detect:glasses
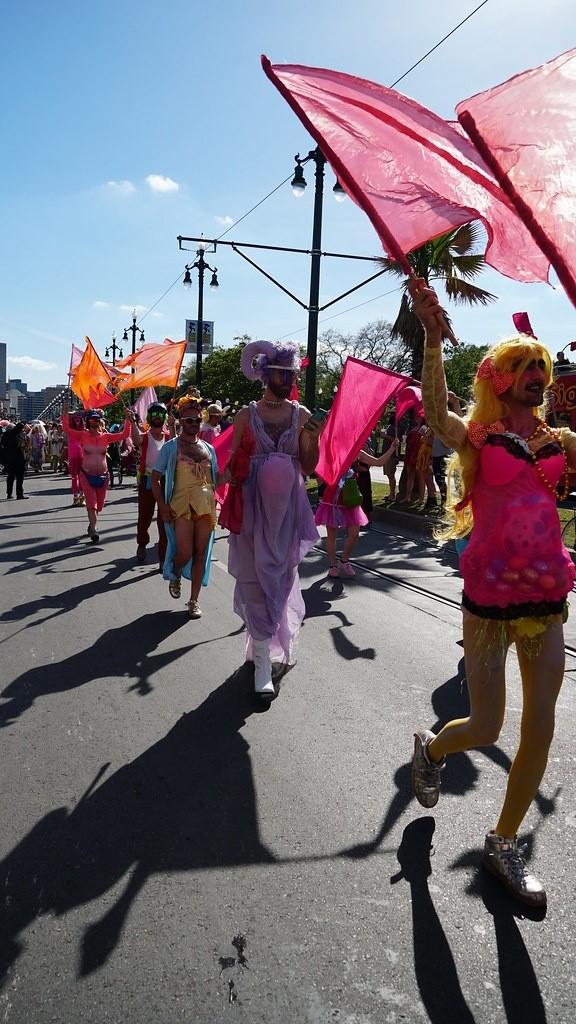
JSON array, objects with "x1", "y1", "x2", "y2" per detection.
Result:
[
  {"x1": 151, "y1": 412, "x2": 165, "y2": 418},
  {"x1": 182, "y1": 417, "x2": 203, "y2": 424}
]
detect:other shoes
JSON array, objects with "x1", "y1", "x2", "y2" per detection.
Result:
[
  {"x1": 17, "y1": 495, "x2": 28, "y2": 499},
  {"x1": 7, "y1": 495, "x2": 13, "y2": 499},
  {"x1": 383, "y1": 495, "x2": 445, "y2": 510},
  {"x1": 91, "y1": 533, "x2": 99, "y2": 542},
  {"x1": 73, "y1": 497, "x2": 80, "y2": 506},
  {"x1": 87, "y1": 526, "x2": 94, "y2": 533},
  {"x1": 79, "y1": 495, "x2": 86, "y2": 505},
  {"x1": 137, "y1": 545, "x2": 146, "y2": 561}
]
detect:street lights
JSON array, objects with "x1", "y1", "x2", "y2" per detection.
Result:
[
  {"x1": 37, "y1": 387, "x2": 79, "y2": 421},
  {"x1": 104, "y1": 331, "x2": 123, "y2": 366},
  {"x1": 290, "y1": 145, "x2": 347, "y2": 410},
  {"x1": 182, "y1": 232, "x2": 220, "y2": 384},
  {"x1": 122, "y1": 309, "x2": 145, "y2": 405}
]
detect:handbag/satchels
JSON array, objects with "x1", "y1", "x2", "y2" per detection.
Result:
[
  {"x1": 82, "y1": 468, "x2": 108, "y2": 486},
  {"x1": 341, "y1": 478, "x2": 363, "y2": 508}
]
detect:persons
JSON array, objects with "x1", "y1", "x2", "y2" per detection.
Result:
[
  {"x1": 410, "y1": 277, "x2": 576, "y2": 903},
  {"x1": 0, "y1": 390, "x2": 479, "y2": 511},
  {"x1": 151, "y1": 394, "x2": 228, "y2": 619},
  {"x1": 62, "y1": 402, "x2": 175, "y2": 572},
  {"x1": 224, "y1": 340, "x2": 324, "y2": 700},
  {"x1": 313, "y1": 437, "x2": 400, "y2": 579},
  {"x1": 553, "y1": 351, "x2": 570, "y2": 365}
]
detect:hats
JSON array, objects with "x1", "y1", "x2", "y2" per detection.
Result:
[{"x1": 207, "y1": 405, "x2": 225, "y2": 416}]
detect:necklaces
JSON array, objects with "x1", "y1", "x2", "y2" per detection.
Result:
[
  {"x1": 262, "y1": 395, "x2": 287, "y2": 409},
  {"x1": 505, "y1": 415, "x2": 568, "y2": 501},
  {"x1": 178, "y1": 434, "x2": 199, "y2": 445}
]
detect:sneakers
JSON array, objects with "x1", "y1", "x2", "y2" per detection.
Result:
[
  {"x1": 481, "y1": 830, "x2": 547, "y2": 905},
  {"x1": 169, "y1": 577, "x2": 181, "y2": 598},
  {"x1": 411, "y1": 727, "x2": 446, "y2": 808},
  {"x1": 329, "y1": 566, "x2": 339, "y2": 577},
  {"x1": 339, "y1": 559, "x2": 355, "y2": 576},
  {"x1": 185, "y1": 599, "x2": 201, "y2": 617}
]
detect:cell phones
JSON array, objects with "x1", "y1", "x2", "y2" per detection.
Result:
[{"x1": 303, "y1": 408, "x2": 328, "y2": 431}]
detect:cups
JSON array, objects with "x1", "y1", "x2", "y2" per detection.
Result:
[{"x1": 419, "y1": 426, "x2": 427, "y2": 433}]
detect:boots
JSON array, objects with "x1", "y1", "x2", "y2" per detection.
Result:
[{"x1": 252, "y1": 637, "x2": 275, "y2": 694}]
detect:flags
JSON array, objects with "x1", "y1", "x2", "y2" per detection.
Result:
[
  {"x1": 275, "y1": 47, "x2": 576, "y2": 317},
  {"x1": 314, "y1": 358, "x2": 425, "y2": 487},
  {"x1": 70, "y1": 334, "x2": 185, "y2": 409}
]
